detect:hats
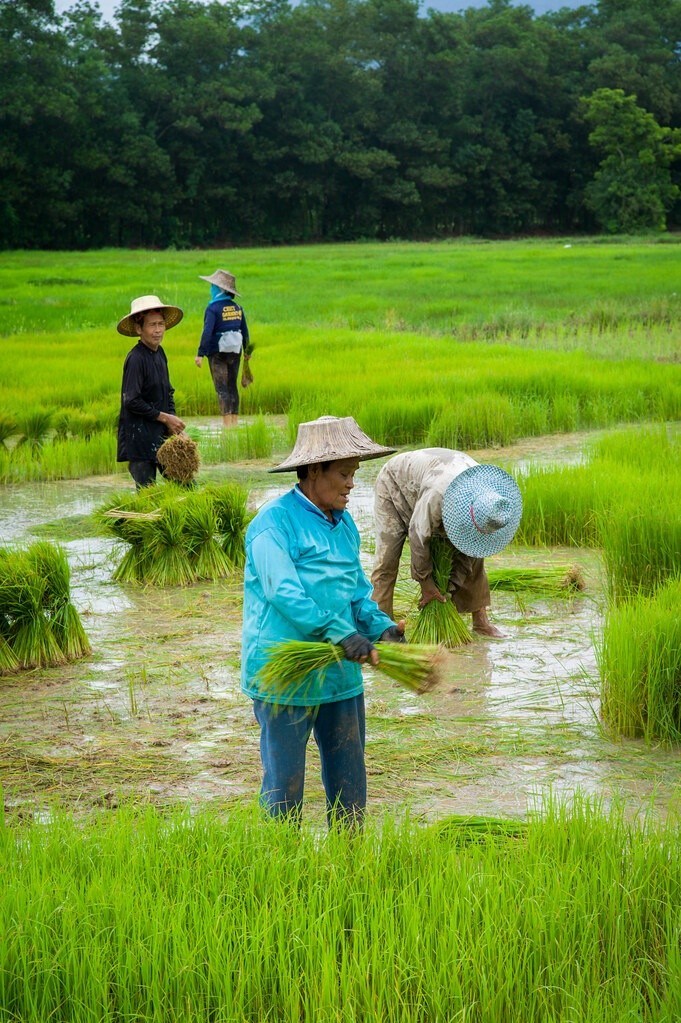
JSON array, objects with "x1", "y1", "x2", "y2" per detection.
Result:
[
  {"x1": 267, "y1": 415, "x2": 397, "y2": 474},
  {"x1": 117, "y1": 295, "x2": 183, "y2": 337},
  {"x1": 198, "y1": 269, "x2": 242, "y2": 299},
  {"x1": 441, "y1": 465, "x2": 522, "y2": 559}
]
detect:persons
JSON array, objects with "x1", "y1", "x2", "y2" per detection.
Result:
[
  {"x1": 371, "y1": 447, "x2": 523, "y2": 637},
  {"x1": 195, "y1": 270, "x2": 252, "y2": 427},
  {"x1": 240, "y1": 416, "x2": 409, "y2": 871},
  {"x1": 116, "y1": 295, "x2": 199, "y2": 496}
]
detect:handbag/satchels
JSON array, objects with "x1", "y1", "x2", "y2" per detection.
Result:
[{"x1": 218, "y1": 329, "x2": 243, "y2": 353}]
